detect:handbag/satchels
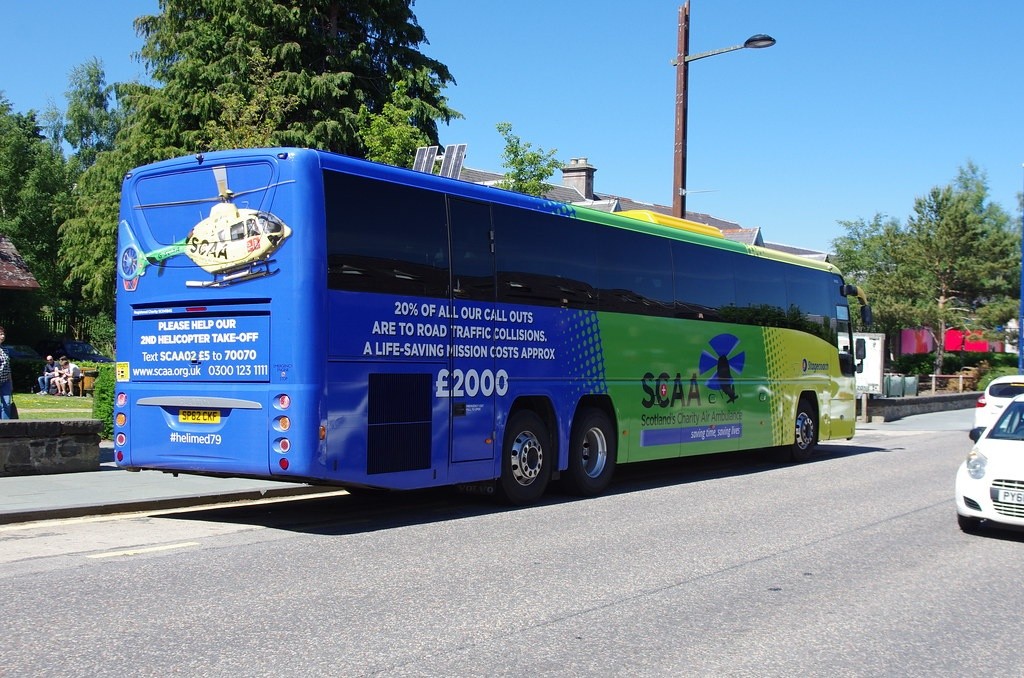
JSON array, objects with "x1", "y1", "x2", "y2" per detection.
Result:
[{"x1": 4, "y1": 402, "x2": 18, "y2": 419}]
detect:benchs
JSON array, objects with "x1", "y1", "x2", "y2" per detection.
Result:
[
  {"x1": 928, "y1": 374, "x2": 974, "y2": 395},
  {"x1": 73, "y1": 370, "x2": 85, "y2": 397}
]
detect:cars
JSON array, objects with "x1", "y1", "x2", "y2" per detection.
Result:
[
  {"x1": 975, "y1": 374, "x2": 1024, "y2": 446},
  {"x1": 954, "y1": 394, "x2": 1024, "y2": 534}
]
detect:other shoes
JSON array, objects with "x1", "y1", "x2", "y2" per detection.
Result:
[
  {"x1": 36, "y1": 391, "x2": 43, "y2": 395},
  {"x1": 55, "y1": 393, "x2": 62, "y2": 396},
  {"x1": 66, "y1": 392, "x2": 73, "y2": 397},
  {"x1": 62, "y1": 391, "x2": 67, "y2": 396},
  {"x1": 40, "y1": 391, "x2": 47, "y2": 395}
]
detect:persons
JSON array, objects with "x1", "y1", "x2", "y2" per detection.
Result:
[
  {"x1": 0, "y1": 326, "x2": 13, "y2": 419},
  {"x1": 50, "y1": 357, "x2": 68, "y2": 396},
  {"x1": 60, "y1": 361, "x2": 80, "y2": 397},
  {"x1": 36, "y1": 356, "x2": 62, "y2": 395}
]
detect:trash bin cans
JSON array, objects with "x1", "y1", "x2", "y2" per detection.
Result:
[{"x1": 883, "y1": 373, "x2": 919, "y2": 397}]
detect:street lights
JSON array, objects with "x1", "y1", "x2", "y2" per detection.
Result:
[{"x1": 671, "y1": 32, "x2": 778, "y2": 222}]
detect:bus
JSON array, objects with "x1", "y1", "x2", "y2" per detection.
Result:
[{"x1": 109, "y1": 146, "x2": 874, "y2": 505}]
